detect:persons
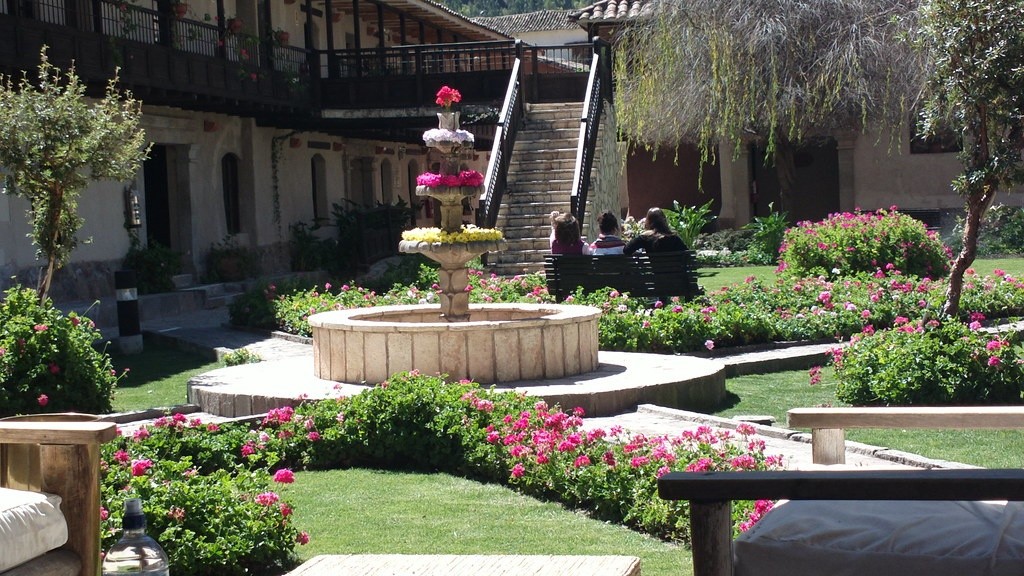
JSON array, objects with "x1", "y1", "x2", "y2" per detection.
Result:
[
  {"x1": 549, "y1": 211, "x2": 589, "y2": 256},
  {"x1": 587, "y1": 210, "x2": 659, "y2": 305},
  {"x1": 623, "y1": 207, "x2": 693, "y2": 308}
]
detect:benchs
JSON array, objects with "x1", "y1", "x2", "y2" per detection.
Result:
[{"x1": 542, "y1": 250, "x2": 706, "y2": 304}]
